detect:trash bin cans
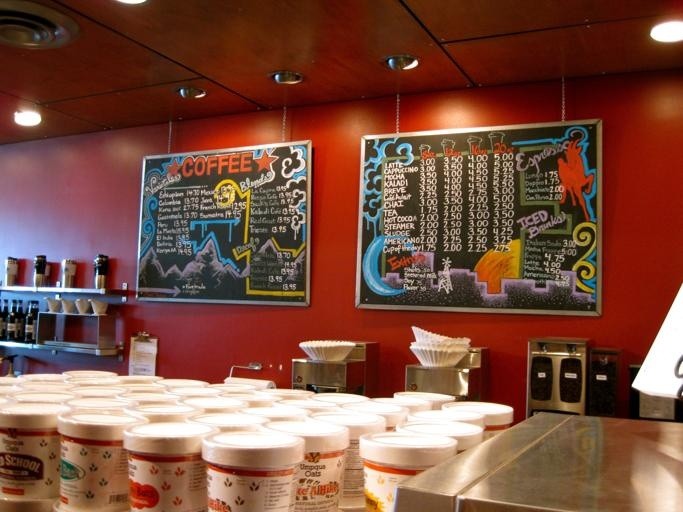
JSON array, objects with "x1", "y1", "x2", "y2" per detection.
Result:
[{"x1": 587, "y1": 350, "x2": 618, "y2": 416}]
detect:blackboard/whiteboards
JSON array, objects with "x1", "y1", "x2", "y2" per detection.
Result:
[
  {"x1": 135, "y1": 139, "x2": 313, "y2": 307},
  {"x1": 354, "y1": 118, "x2": 603, "y2": 318}
]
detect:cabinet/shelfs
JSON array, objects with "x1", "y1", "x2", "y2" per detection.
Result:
[{"x1": 0, "y1": 284, "x2": 127, "y2": 357}]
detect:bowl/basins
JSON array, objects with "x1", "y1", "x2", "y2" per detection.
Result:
[
  {"x1": 298, "y1": 340, "x2": 355, "y2": 363},
  {"x1": 43, "y1": 297, "x2": 110, "y2": 315},
  {"x1": 406, "y1": 325, "x2": 471, "y2": 368}
]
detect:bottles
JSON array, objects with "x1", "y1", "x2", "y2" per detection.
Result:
[{"x1": 0, "y1": 299, "x2": 39, "y2": 344}]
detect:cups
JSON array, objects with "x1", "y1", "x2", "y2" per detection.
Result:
[
  {"x1": 61, "y1": 258, "x2": 76, "y2": 288},
  {"x1": 33, "y1": 255, "x2": 50, "y2": 287},
  {"x1": 93, "y1": 253, "x2": 109, "y2": 290},
  {"x1": 4, "y1": 257, "x2": 17, "y2": 286}
]
